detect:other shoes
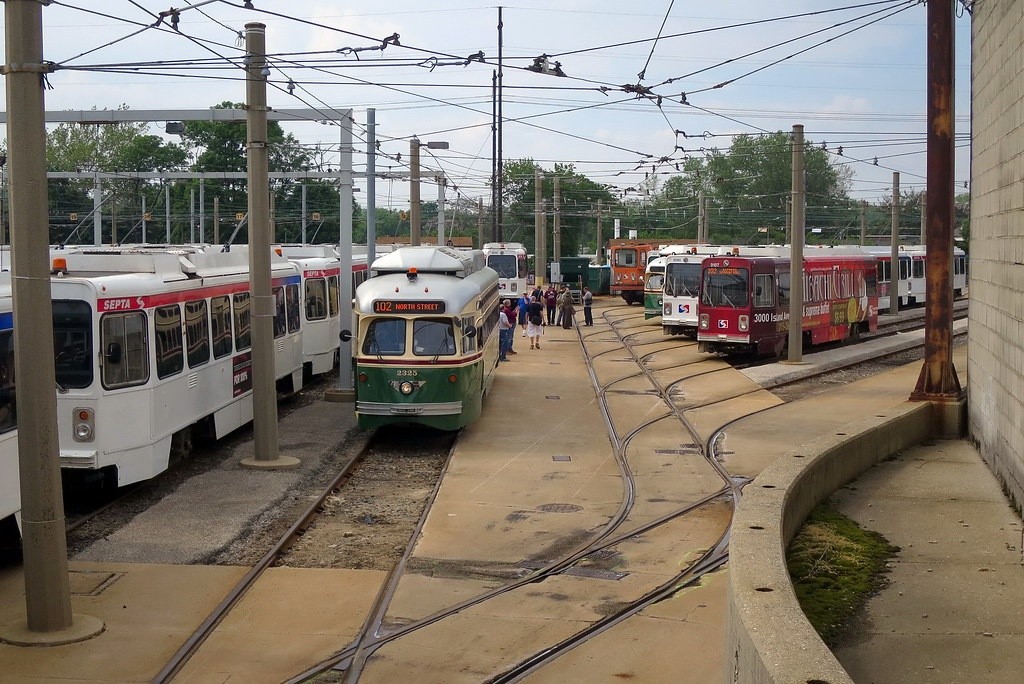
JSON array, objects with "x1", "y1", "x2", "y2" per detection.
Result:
[
  {"x1": 556, "y1": 323, "x2": 562, "y2": 326},
  {"x1": 500, "y1": 358, "x2": 510, "y2": 362},
  {"x1": 547, "y1": 320, "x2": 555, "y2": 325},
  {"x1": 506, "y1": 349, "x2": 517, "y2": 355},
  {"x1": 582, "y1": 323, "x2": 594, "y2": 326},
  {"x1": 529, "y1": 344, "x2": 534, "y2": 350},
  {"x1": 522, "y1": 330, "x2": 526, "y2": 337},
  {"x1": 564, "y1": 326, "x2": 571, "y2": 329},
  {"x1": 535, "y1": 342, "x2": 540, "y2": 350}
]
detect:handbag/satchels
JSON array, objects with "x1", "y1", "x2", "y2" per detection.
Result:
[
  {"x1": 585, "y1": 298, "x2": 592, "y2": 306},
  {"x1": 571, "y1": 307, "x2": 576, "y2": 315},
  {"x1": 531, "y1": 315, "x2": 542, "y2": 326}
]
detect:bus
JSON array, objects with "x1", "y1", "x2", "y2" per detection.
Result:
[
  {"x1": 608, "y1": 244, "x2": 965, "y2": 343},
  {"x1": 481, "y1": 240, "x2": 527, "y2": 304},
  {"x1": 696, "y1": 247, "x2": 879, "y2": 360},
  {"x1": 0, "y1": 241, "x2": 398, "y2": 547},
  {"x1": 340, "y1": 244, "x2": 502, "y2": 430}
]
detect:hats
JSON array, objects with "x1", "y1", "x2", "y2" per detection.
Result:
[
  {"x1": 548, "y1": 291, "x2": 555, "y2": 295},
  {"x1": 564, "y1": 291, "x2": 572, "y2": 297},
  {"x1": 522, "y1": 290, "x2": 528, "y2": 295}
]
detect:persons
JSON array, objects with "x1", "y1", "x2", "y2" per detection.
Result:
[
  {"x1": 544, "y1": 286, "x2": 557, "y2": 326},
  {"x1": 525, "y1": 296, "x2": 546, "y2": 349},
  {"x1": 503, "y1": 299, "x2": 517, "y2": 354},
  {"x1": 556, "y1": 285, "x2": 575, "y2": 329},
  {"x1": 532, "y1": 285, "x2": 544, "y2": 311},
  {"x1": 582, "y1": 287, "x2": 593, "y2": 326},
  {"x1": 516, "y1": 291, "x2": 531, "y2": 337},
  {"x1": 499, "y1": 304, "x2": 513, "y2": 362}
]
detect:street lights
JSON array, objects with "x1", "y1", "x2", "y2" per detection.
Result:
[{"x1": 411, "y1": 139, "x2": 450, "y2": 247}]
